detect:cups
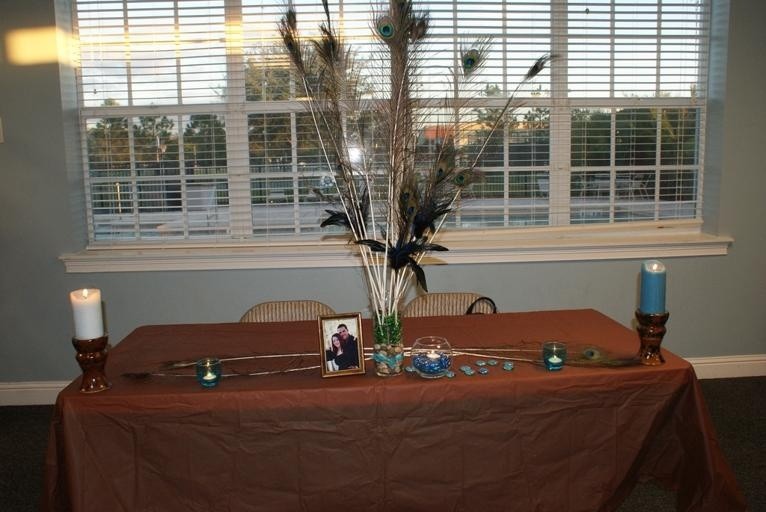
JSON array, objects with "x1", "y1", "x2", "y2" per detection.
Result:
[
  {"x1": 195, "y1": 358, "x2": 220, "y2": 387},
  {"x1": 542, "y1": 342, "x2": 566, "y2": 372}
]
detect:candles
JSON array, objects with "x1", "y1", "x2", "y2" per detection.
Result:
[
  {"x1": 69, "y1": 289, "x2": 103, "y2": 341},
  {"x1": 640, "y1": 259, "x2": 667, "y2": 315}
]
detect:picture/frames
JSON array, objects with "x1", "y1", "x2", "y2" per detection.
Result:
[{"x1": 316, "y1": 311, "x2": 367, "y2": 376}]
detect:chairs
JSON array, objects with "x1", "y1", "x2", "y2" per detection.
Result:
[
  {"x1": 403, "y1": 294, "x2": 491, "y2": 318},
  {"x1": 238, "y1": 301, "x2": 335, "y2": 323}
]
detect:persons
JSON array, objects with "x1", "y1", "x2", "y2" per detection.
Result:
[{"x1": 326, "y1": 324, "x2": 359, "y2": 370}]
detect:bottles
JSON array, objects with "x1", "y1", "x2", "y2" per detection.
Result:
[{"x1": 412, "y1": 336, "x2": 451, "y2": 378}]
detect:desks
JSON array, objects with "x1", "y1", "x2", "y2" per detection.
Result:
[{"x1": 50, "y1": 309, "x2": 740, "y2": 511}]
